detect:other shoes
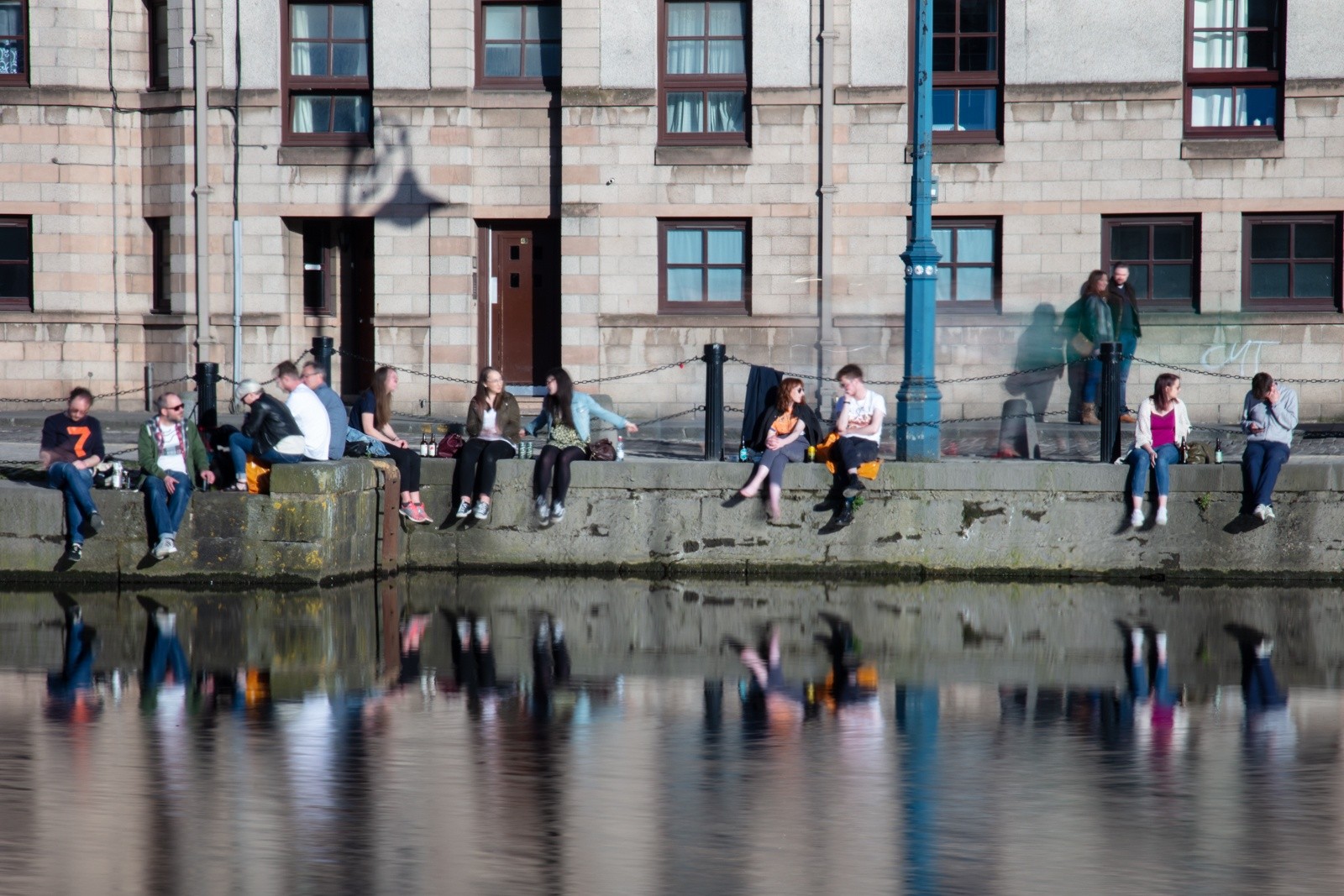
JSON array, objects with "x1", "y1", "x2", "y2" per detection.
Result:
[
  {"x1": 150, "y1": 540, "x2": 177, "y2": 559},
  {"x1": 1119, "y1": 412, "x2": 1136, "y2": 422},
  {"x1": 67, "y1": 545, "x2": 82, "y2": 561},
  {"x1": 536, "y1": 495, "x2": 549, "y2": 525},
  {"x1": 843, "y1": 481, "x2": 867, "y2": 499},
  {"x1": 222, "y1": 483, "x2": 249, "y2": 491},
  {"x1": 1155, "y1": 509, "x2": 1167, "y2": 526},
  {"x1": 90, "y1": 512, "x2": 105, "y2": 534},
  {"x1": 549, "y1": 500, "x2": 564, "y2": 523},
  {"x1": 1131, "y1": 509, "x2": 1145, "y2": 527},
  {"x1": 834, "y1": 508, "x2": 854, "y2": 526}
]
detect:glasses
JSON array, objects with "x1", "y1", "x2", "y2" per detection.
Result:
[
  {"x1": 792, "y1": 388, "x2": 803, "y2": 393},
  {"x1": 547, "y1": 378, "x2": 556, "y2": 385},
  {"x1": 165, "y1": 403, "x2": 184, "y2": 411}
]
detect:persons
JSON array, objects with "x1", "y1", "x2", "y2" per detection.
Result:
[
  {"x1": 301, "y1": 362, "x2": 348, "y2": 462},
  {"x1": 1107, "y1": 262, "x2": 1142, "y2": 423},
  {"x1": 726, "y1": 611, "x2": 883, "y2": 768},
  {"x1": 225, "y1": 380, "x2": 306, "y2": 492},
  {"x1": 1115, "y1": 373, "x2": 1191, "y2": 528},
  {"x1": 273, "y1": 361, "x2": 331, "y2": 462},
  {"x1": 739, "y1": 377, "x2": 823, "y2": 514},
  {"x1": 1245, "y1": 639, "x2": 1297, "y2": 764},
  {"x1": 829, "y1": 364, "x2": 886, "y2": 526},
  {"x1": 1077, "y1": 270, "x2": 1115, "y2": 425},
  {"x1": 454, "y1": 367, "x2": 521, "y2": 520},
  {"x1": 138, "y1": 392, "x2": 216, "y2": 559},
  {"x1": 348, "y1": 366, "x2": 434, "y2": 525},
  {"x1": 39, "y1": 386, "x2": 105, "y2": 562},
  {"x1": 1240, "y1": 372, "x2": 1298, "y2": 524},
  {"x1": 1132, "y1": 628, "x2": 1189, "y2": 758},
  {"x1": 397, "y1": 610, "x2": 572, "y2": 722},
  {"x1": 46, "y1": 605, "x2": 272, "y2": 731},
  {"x1": 521, "y1": 368, "x2": 638, "y2": 526}
]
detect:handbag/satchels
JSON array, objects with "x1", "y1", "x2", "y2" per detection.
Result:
[
  {"x1": 1073, "y1": 332, "x2": 1094, "y2": 357},
  {"x1": 345, "y1": 426, "x2": 390, "y2": 456},
  {"x1": 438, "y1": 433, "x2": 465, "y2": 457},
  {"x1": 587, "y1": 439, "x2": 616, "y2": 461},
  {"x1": 244, "y1": 452, "x2": 270, "y2": 493},
  {"x1": 1185, "y1": 442, "x2": 1214, "y2": 464}
]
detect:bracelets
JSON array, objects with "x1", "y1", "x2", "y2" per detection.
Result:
[
  {"x1": 393, "y1": 437, "x2": 398, "y2": 440},
  {"x1": 843, "y1": 401, "x2": 850, "y2": 404}
]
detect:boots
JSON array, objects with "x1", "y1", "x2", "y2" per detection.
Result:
[{"x1": 1080, "y1": 401, "x2": 1100, "y2": 425}]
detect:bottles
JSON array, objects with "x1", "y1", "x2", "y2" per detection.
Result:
[
  {"x1": 616, "y1": 435, "x2": 625, "y2": 460},
  {"x1": 1216, "y1": 438, "x2": 1223, "y2": 464},
  {"x1": 428, "y1": 433, "x2": 437, "y2": 457},
  {"x1": 739, "y1": 435, "x2": 747, "y2": 462},
  {"x1": 1181, "y1": 436, "x2": 1188, "y2": 464},
  {"x1": 420, "y1": 433, "x2": 428, "y2": 458},
  {"x1": 808, "y1": 446, "x2": 815, "y2": 463}
]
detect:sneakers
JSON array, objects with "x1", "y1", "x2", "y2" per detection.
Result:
[
  {"x1": 456, "y1": 501, "x2": 472, "y2": 517},
  {"x1": 475, "y1": 502, "x2": 489, "y2": 520},
  {"x1": 1254, "y1": 504, "x2": 1275, "y2": 524},
  {"x1": 415, "y1": 502, "x2": 433, "y2": 524},
  {"x1": 398, "y1": 502, "x2": 427, "y2": 523}
]
